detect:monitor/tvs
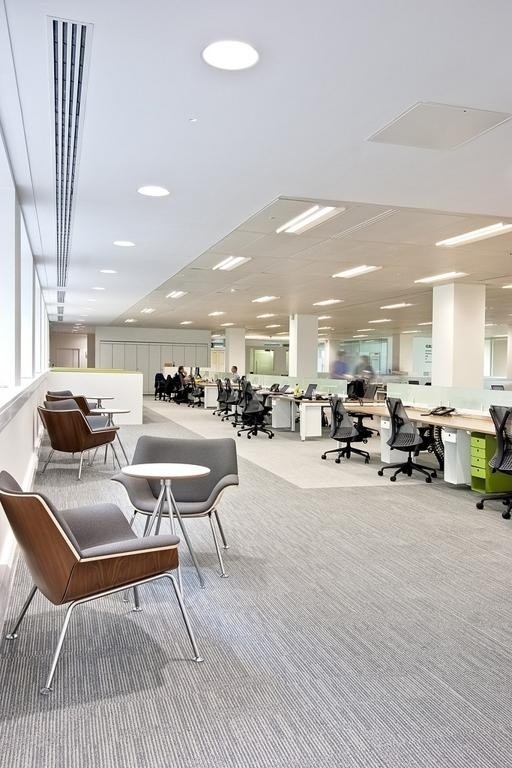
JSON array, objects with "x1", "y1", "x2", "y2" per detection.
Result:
[{"x1": 190, "y1": 366, "x2": 201, "y2": 379}]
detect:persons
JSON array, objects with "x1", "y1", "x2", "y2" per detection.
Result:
[
  {"x1": 354, "y1": 353, "x2": 375, "y2": 374},
  {"x1": 174, "y1": 365, "x2": 188, "y2": 388},
  {"x1": 329, "y1": 348, "x2": 351, "y2": 379},
  {"x1": 228, "y1": 364, "x2": 240, "y2": 391}
]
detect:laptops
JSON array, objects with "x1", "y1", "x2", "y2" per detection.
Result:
[
  {"x1": 270, "y1": 385, "x2": 290, "y2": 395},
  {"x1": 294, "y1": 384, "x2": 317, "y2": 400}
]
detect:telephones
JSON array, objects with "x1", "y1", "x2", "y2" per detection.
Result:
[
  {"x1": 270, "y1": 383, "x2": 279, "y2": 392},
  {"x1": 431, "y1": 407, "x2": 455, "y2": 416},
  {"x1": 309, "y1": 394, "x2": 321, "y2": 400}
]
